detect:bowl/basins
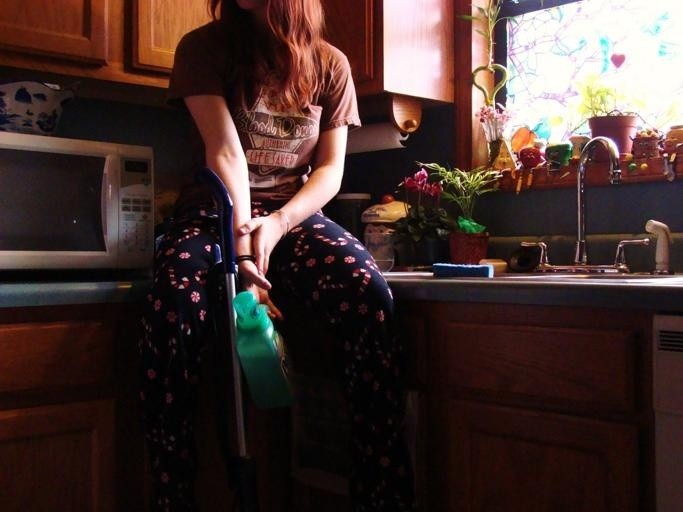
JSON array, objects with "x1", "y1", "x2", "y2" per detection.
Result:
[{"x1": 0, "y1": 82, "x2": 76, "y2": 134}]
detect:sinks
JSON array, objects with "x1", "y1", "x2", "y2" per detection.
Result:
[{"x1": 570, "y1": 272, "x2": 673, "y2": 281}]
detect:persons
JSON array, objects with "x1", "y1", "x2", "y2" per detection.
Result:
[{"x1": 136, "y1": 0, "x2": 417, "y2": 510}]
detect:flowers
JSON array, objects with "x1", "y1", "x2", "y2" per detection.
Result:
[
  {"x1": 393, "y1": 164, "x2": 449, "y2": 240},
  {"x1": 477, "y1": 103, "x2": 511, "y2": 121}
]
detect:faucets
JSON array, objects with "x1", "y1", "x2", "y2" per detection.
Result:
[{"x1": 520, "y1": 136, "x2": 651, "y2": 272}]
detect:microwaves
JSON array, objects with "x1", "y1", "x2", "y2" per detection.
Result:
[{"x1": 0, "y1": 132, "x2": 157, "y2": 272}]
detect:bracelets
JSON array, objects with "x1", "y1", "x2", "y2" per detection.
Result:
[
  {"x1": 269, "y1": 209, "x2": 290, "y2": 235},
  {"x1": 234, "y1": 257, "x2": 255, "y2": 266},
  {"x1": 232, "y1": 254, "x2": 256, "y2": 262}
]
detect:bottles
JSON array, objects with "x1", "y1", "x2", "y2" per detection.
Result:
[{"x1": 334, "y1": 192, "x2": 371, "y2": 243}]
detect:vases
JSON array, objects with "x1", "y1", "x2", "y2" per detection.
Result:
[
  {"x1": 483, "y1": 119, "x2": 505, "y2": 167},
  {"x1": 415, "y1": 239, "x2": 445, "y2": 266}
]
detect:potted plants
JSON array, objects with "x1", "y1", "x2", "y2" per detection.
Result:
[
  {"x1": 417, "y1": 161, "x2": 504, "y2": 265},
  {"x1": 559, "y1": 79, "x2": 676, "y2": 153}
]
